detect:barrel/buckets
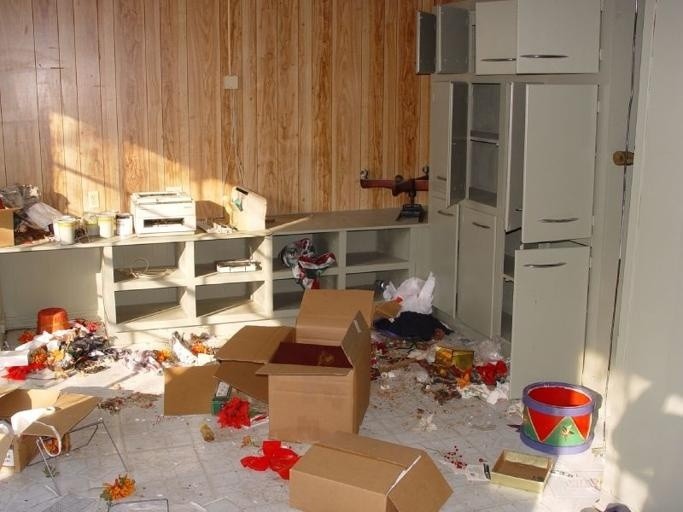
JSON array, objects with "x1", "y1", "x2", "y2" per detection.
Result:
[{"x1": 37, "y1": 308, "x2": 71, "y2": 330}]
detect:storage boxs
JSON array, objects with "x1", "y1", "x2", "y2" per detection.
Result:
[
  {"x1": 1, "y1": 388, "x2": 104, "y2": 472},
  {"x1": 163, "y1": 326, "x2": 268, "y2": 416},
  {"x1": 289, "y1": 430, "x2": 452, "y2": 511},
  {"x1": 214, "y1": 289, "x2": 402, "y2": 446}
]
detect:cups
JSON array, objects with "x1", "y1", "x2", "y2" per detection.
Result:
[
  {"x1": 52, "y1": 218, "x2": 76, "y2": 246},
  {"x1": 116, "y1": 215, "x2": 134, "y2": 235},
  {"x1": 97, "y1": 217, "x2": 114, "y2": 237},
  {"x1": 86, "y1": 223, "x2": 98, "y2": 235}
]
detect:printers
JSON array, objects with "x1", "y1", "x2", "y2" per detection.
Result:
[{"x1": 130, "y1": 188, "x2": 197, "y2": 238}]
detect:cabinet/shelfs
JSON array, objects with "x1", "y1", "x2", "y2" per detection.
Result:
[{"x1": 1, "y1": 0, "x2": 642, "y2": 399}]
